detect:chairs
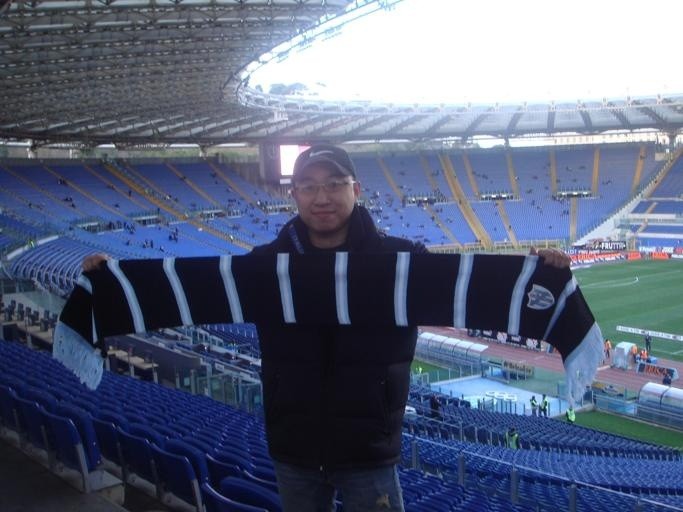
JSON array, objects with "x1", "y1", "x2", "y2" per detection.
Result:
[{"x1": 0, "y1": 337, "x2": 681, "y2": 512}]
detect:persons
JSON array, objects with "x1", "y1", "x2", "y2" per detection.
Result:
[
  {"x1": 57, "y1": 170, "x2": 294, "y2": 253},
  {"x1": 633, "y1": 333, "x2": 652, "y2": 363},
  {"x1": 566, "y1": 405, "x2": 576, "y2": 425},
  {"x1": 604, "y1": 339, "x2": 612, "y2": 358},
  {"x1": 504, "y1": 428, "x2": 521, "y2": 451},
  {"x1": 662, "y1": 374, "x2": 669, "y2": 386},
  {"x1": 530, "y1": 394, "x2": 548, "y2": 418},
  {"x1": 361, "y1": 162, "x2": 612, "y2": 245},
  {"x1": 82, "y1": 145, "x2": 572, "y2": 512},
  {"x1": 429, "y1": 394, "x2": 441, "y2": 418}
]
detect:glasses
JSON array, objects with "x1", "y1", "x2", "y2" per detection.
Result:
[{"x1": 291, "y1": 179, "x2": 358, "y2": 191}]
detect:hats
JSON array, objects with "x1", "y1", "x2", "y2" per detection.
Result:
[{"x1": 293, "y1": 144, "x2": 356, "y2": 177}]
